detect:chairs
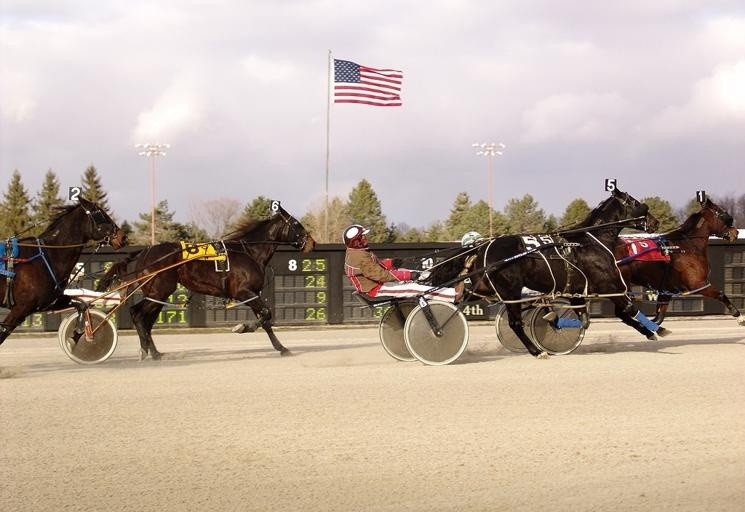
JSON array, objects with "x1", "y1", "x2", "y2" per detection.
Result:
[{"x1": 352, "y1": 290, "x2": 405, "y2": 330}]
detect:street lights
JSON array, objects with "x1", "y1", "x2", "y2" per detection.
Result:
[
  {"x1": 471, "y1": 143, "x2": 505, "y2": 236},
  {"x1": 132, "y1": 142, "x2": 173, "y2": 245}
]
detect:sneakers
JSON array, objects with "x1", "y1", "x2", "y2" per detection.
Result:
[
  {"x1": 455, "y1": 281, "x2": 465, "y2": 302},
  {"x1": 119, "y1": 284, "x2": 127, "y2": 303}
]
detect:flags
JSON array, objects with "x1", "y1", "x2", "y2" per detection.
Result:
[{"x1": 335, "y1": 59, "x2": 404, "y2": 108}]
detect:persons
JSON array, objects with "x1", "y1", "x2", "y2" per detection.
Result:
[
  {"x1": 342, "y1": 223, "x2": 472, "y2": 306},
  {"x1": 63, "y1": 283, "x2": 130, "y2": 306},
  {"x1": 459, "y1": 229, "x2": 543, "y2": 296}
]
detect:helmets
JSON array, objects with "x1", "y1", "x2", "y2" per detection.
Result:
[
  {"x1": 461, "y1": 231, "x2": 481, "y2": 248},
  {"x1": 342, "y1": 225, "x2": 370, "y2": 248}
]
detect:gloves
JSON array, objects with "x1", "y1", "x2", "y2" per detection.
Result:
[
  {"x1": 410, "y1": 271, "x2": 420, "y2": 281},
  {"x1": 393, "y1": 259, "x2": 403, "y2": 267}
]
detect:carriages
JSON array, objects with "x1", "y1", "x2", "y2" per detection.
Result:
[
  {"x1": 36, "y1": 205, "x2": 317, "y2": 366},
  {"x1": 352, "y1": 188, "x2": 674, "y2": 367},
  {"x1": 493, "y1": 187, "x2": 744, "y2": 367}
]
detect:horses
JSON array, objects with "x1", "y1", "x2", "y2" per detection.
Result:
[
  {"x1": 611, "y1": 198, "x2": 745, "y2": 327},
  {"x1": 92, "y1": 204, "x2": 316, "y2": 361},
  {"x1": 0, "y1": 194, "x2": 129, "y2": 347},
  {"x1": 431, "y1": 186, "x2": 673, "y2": 360}
]
